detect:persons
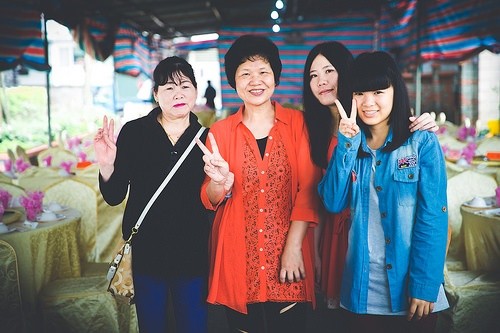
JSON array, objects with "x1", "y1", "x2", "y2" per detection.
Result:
[
  {"x1": 195, "y1": 34, "x2": 320, "y2": 333},
  {"x1": 316, "y1": 50, "x2": 452, "y2": 333},
  {"x1": 302, "y1": 39, "x2": 441, "y2": 332},
  {"x1": 202, "y1": 78, "x2": 217, "y2": 109},
  {"x1": 91, "y1": 55, "x2": 235, "y2": 333}
]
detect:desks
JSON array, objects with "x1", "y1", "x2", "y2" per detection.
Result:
[
  {"x1": 460, "y1": 197, "x2": 500, "y2": 271},
  {"x1": 0, "y1": 208, "x2": 97, "y2": 292}
]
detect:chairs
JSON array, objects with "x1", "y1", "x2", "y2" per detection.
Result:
[
  {"x1": 0, "y1": 179, "x2": 97, "y2": 233},
  {"x1": 444, "y1": 170, "x2": 500, "y2": 333}
]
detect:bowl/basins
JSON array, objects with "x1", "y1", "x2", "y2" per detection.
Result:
[
  {"x1": 40, "y1": 211, "x2": 56, "y2": 220},
  {"x1": 469, "y1": 197, "x2": 486, "y2": 206},
  {"x1": 48, "y1": 202, "x2": 62, "y2": 211},
  {"x1": 0, "y1": 222, "x2": 9, "y2": 233}
]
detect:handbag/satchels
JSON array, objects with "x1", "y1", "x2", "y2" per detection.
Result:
[{"x1": 105, "y1": 240, "x2": 135, "y2": 306}]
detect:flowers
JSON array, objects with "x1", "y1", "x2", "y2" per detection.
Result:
[{"x1": 0, "y1": 153, "x2": 86, "y2": 221}]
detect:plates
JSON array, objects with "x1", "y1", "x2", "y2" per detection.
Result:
[
  {"x1": 463, "y1": 200, "x2": 495, "y2": 208},
  {"x1": 488, "y1": 208, "x2": 500, "y2": 216},
  {"x1": 36, "y1": 214, "x2": 66, "y2": 222},
  {"x1": 0, "y1": 226, "x2": 16, "y2": 234},
  {"x1": 42, "y1": 206, "x2": 68, "y2": 213}
]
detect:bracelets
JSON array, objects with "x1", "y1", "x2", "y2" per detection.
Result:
[{"x1": 224, "y1": 194, "x2": 232, "y2": 198}]
